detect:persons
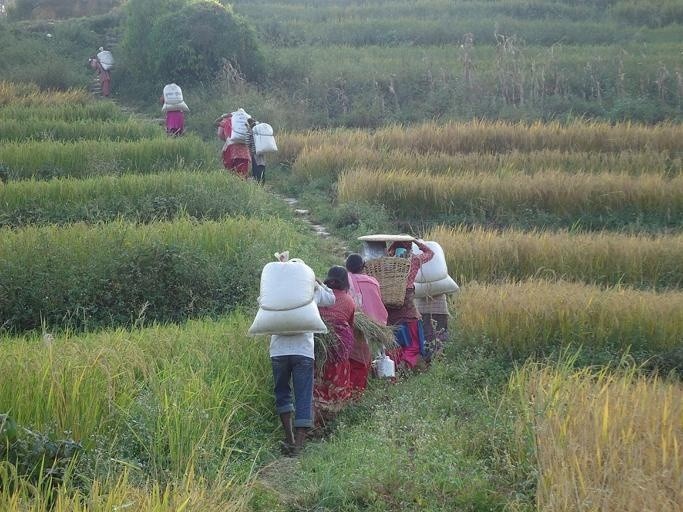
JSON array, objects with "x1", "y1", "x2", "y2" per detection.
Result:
[
  {"x1": 381, "y1": 232, "x2": 435, "y2": 385},
  {"x1": 416, "y1": 294, "x2": 450, "y2": 359},
  {"x1": 245, "y1": 117, "x2": 267, "y2": 187},
  {"x1": 160, "y1": 95, "x2": 185, "y2": 135},
  {"x1": 213, "y1": 113, "x2": 252, "y2": 181},
  {"x1": 89, "y1": 55, "x2": 111, "y2": 97},
  {"x1": 311, "y1": 264, "x2": 356, "y2": 428},
  {"x1": 269, "y1": 276, "x2": 336, "y2": 458},
  {"x1": 345, "y1": 254, "x2": 388, "y2": 401}
]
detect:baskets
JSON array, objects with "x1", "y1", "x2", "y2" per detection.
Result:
[{"x1": 363, "y1": 256, "x2": 410, "y2": 308}]
